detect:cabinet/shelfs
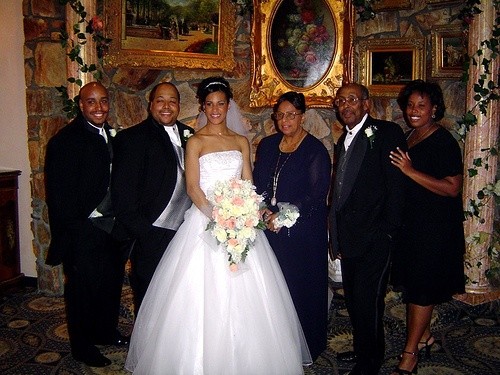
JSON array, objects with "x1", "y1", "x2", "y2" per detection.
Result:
[{"x1": 0, "y1": 170, "x2": 25, "y2": 293}]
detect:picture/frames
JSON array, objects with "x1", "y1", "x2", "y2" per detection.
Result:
[
  {"x1": 103, "y1": 0, "x2": 236, "y2": 71},
  {"x1": 357, "y1": 38, "x2": 426, "y2": 99},
  {"x1": 431, "y1": 24, "x2": 468, "y2": 78},
  {"x1": 249, "y1": 0, "x2": 357, "y2": 110}
]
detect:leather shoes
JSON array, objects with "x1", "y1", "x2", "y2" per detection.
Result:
[
  {"x1": 115, "y1": 336, "x2": 129, "y2": 346},
  {"x1": 349, "y1": 358, "x2": 385, "y2": 375},
  {"x1": 337, "y1": 351, "x2": 357, "y2": 362},
  {"x1": 72, "y1": 350, "x2": 112, "y2": 367}
]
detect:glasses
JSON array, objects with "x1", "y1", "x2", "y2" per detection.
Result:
[
  {"x1": 274, "y1": 111, "x2": 303, "y2": 120},
  {"x1": 334, "y1": 97, "x2": 366, "y2": 107}
]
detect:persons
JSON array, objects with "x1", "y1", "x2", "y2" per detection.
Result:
[
  {"x1": 328, "y1": 81, "x2": 409, "y2": 375},
  {"x1": 44, "y1": 81, "x2": 130, "y2": 367},
  {"x1": 125, "y1": 77, "x2": 314, "y2": 375},
  {"x1": 252, "y1": 91, "x2": 332, "y2": 362},
  {"x1": 109, "y1": 82, "x2": 196, "y2": 322}
]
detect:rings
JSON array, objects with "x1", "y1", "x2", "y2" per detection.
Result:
[{"x1": 400, "y1": 157, "x2": 402, "y2": 160}]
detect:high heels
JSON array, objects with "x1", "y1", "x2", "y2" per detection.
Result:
[
  {"x1": 417, "y1": 335, "x2": 436, "y2": 355},
  {"x1": 394, "y1": 351, "x2": 419, "y2": 375}
]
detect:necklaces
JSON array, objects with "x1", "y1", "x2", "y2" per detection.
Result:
[
  {"x1": 409, "y1": 128, "x2": 432, "y2": 144},
  {"x1": 270, "y1": 134, "x2": 303, "y2": 206},
  {"x1": 390, "y1": 79, "x2": 466, "y2": 375},
  {"x1": 207, "y1": 127, "x2": 225, "y2": 136}
]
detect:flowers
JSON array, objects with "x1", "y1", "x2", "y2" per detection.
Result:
[
  {"x1": 365, "y1": 125, "x2": 378, "y2": 142},
  {"x1": 183, "y1": 129, "x2": 193, "y2": 142},
  {"x1": 109, "y1": 129, "x2": 117, "y2": 137},
  {"x1": 206, "y1": 177, "x2": 268, "y2": 272},
  {"x1": 55, "y1": 0, "x2": 112, "y2": 121}
]
